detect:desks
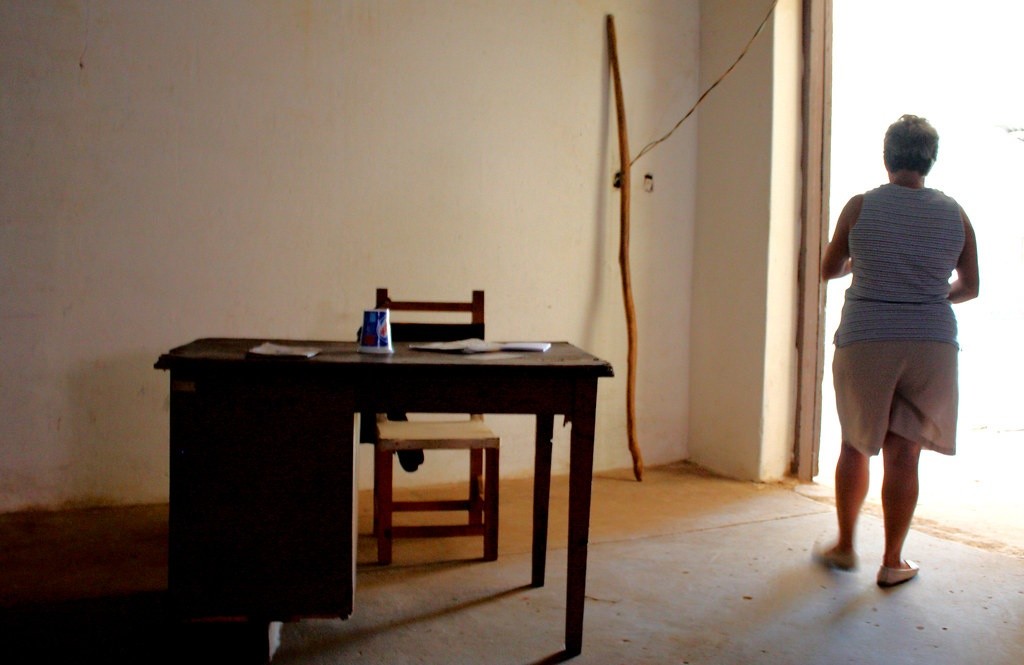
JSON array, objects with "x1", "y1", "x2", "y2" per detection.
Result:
[{"x1": 155, "y1": 337, "x2": 615, "y2": 658}]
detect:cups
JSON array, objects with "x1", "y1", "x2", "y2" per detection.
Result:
[{"x1": 357, "y1": 309, "x2": 393, "y2": 353}]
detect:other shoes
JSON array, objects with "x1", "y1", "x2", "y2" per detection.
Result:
[
  {"x1": 815, "y1": 542, "x2": 856, "y2": 570},
  {"x1": 877, "y1": 559, "x2": 918, "y2": 587}
]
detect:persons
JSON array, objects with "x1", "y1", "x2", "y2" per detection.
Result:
[{"x1": 815, "y1": 115, "x2": 980, "y2": 586}]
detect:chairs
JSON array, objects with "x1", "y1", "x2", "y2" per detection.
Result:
[{"x1": 373, "y1": 287, "x2": 501, "y2": 566}]
